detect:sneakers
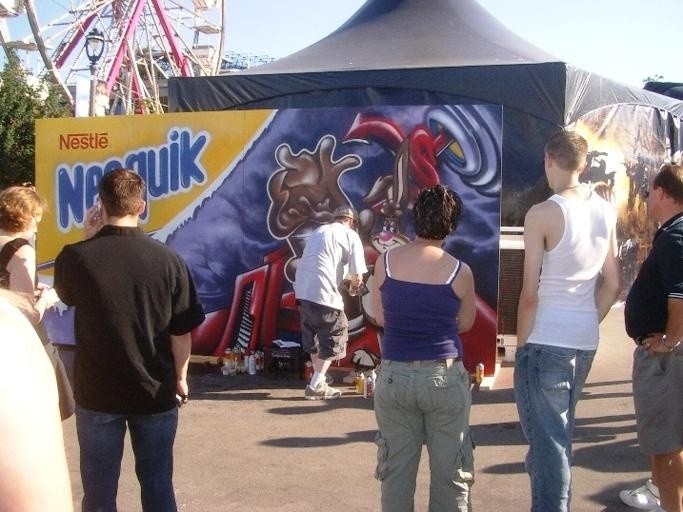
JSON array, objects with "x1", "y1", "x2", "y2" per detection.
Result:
[
  {"x1": 305, "y1": 372, "x2": 341, "y2": 400},
  {"x1": 619, "y1": 479, "x2": 666, "y2": 512}
]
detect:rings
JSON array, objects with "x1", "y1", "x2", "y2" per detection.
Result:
[{"x1": 646, "y1": 342, "x2": 651, "y2": 347}]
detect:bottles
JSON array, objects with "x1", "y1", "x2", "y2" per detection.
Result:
[
  {"x1": 475, "y1": 362, "x2": 484, "y2": 383},
  {"x1": 356, "y1": 371, "x2": 373, "y2": 398},
  {"x1": 304, "y1": 359, "x2": 312, "y2": 381},
  {"x1": 221, "y1": 346, "x2": 265, "y2": 376}
]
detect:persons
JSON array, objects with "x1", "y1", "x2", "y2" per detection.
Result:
[
  {"x1": 54, "y1": 170, "x2": 205, "y2": 512},
  {"x1": 372, "y1": 183, "x2": 475, "y2": 512},
  {"x1": 0, "y1": 186, "x2": 74, "y2": 512},
  {"x1": 294, "y1": 204, "x2": 369, "y2": 401},
  {"x1": 513, "y1": 131, "x2": 620, "y2": 512},
  {"x1": 620, "y1": 163, "x2": 682, "y2": 512}
]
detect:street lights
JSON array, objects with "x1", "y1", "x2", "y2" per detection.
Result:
[{"x1": 84, "y1": 28, "x2": 104, "y2": 117}]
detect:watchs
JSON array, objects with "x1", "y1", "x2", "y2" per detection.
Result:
[{"x1": 661, "y1": 334, "x2": 680, "y2": 350}]
